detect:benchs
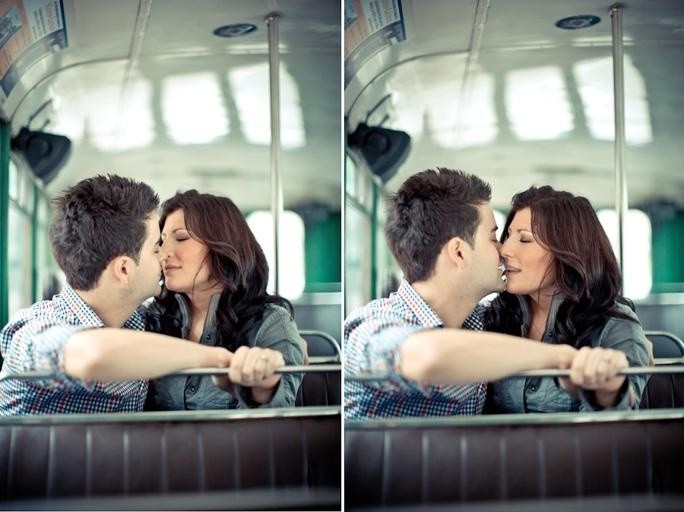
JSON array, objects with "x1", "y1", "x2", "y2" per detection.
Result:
[
  {"x1": 0, "y1": 323, "x2": 341, "y2": 511},
  {"x1": 344, "y1": 331, "x2": 683, "y2": 511}
]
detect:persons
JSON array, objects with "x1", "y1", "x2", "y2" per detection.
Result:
[
  {"x1": 144, "y1": 190, "x2": 306, "y2": 410},
  {"x1": 343, "y1": 166, "x2": 654, "y2": 417},
  {"x1": 484, "y1": 185, "x2": 650, "y2": 414},
  {"x1": 0, "y1": 173, "x2": 308, "y2": 415}
]
